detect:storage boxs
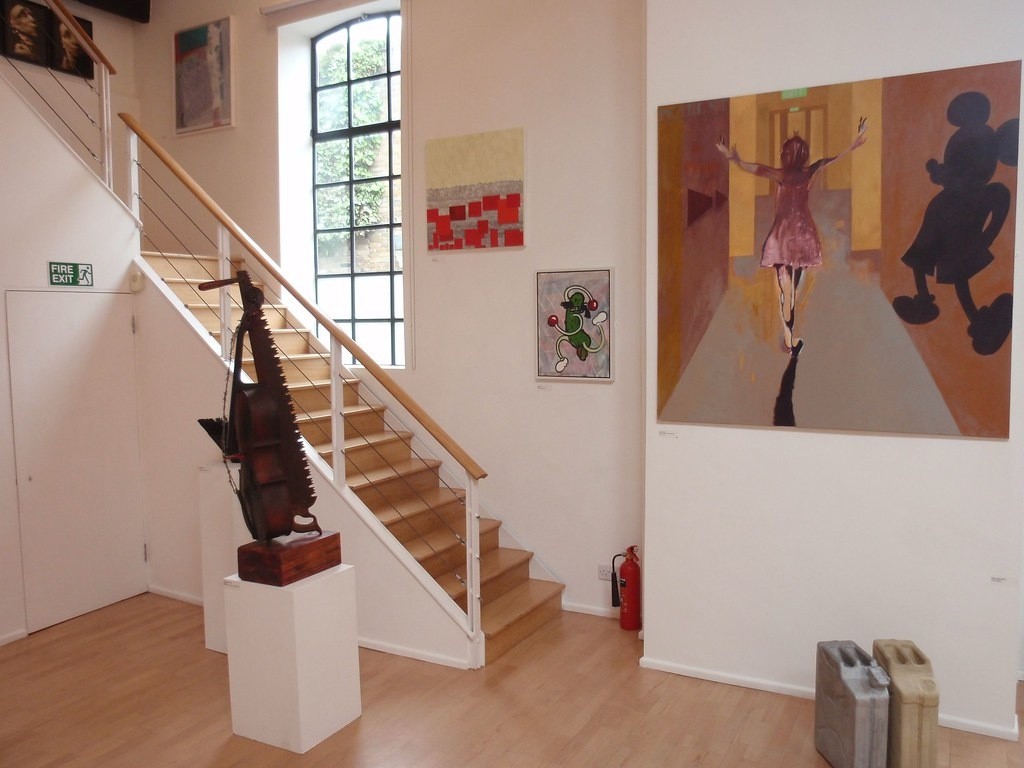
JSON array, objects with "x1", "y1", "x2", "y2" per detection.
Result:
[{"x1": 238, "y1": 530, "x2": 341, "y2": 587}]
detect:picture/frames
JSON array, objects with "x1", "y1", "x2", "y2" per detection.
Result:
[
  {"x1": 0, "y1": 0, "x2": 94, "y2": 80},
  {"x1": 174, "y1": 15, "x2": 236, "y2": 138},
  {"x1": 535, "y1": 266, "x2": 616, "y2": 382}
]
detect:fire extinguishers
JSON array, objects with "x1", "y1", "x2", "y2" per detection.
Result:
[{"x1": 611, "y1": 545, "x2": 641, "y2": 631}]
